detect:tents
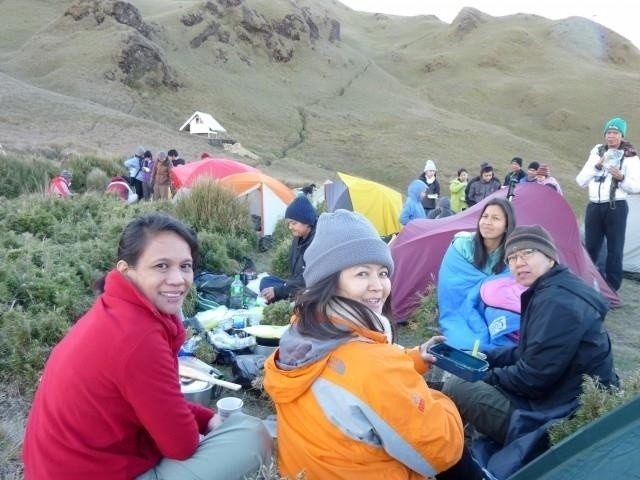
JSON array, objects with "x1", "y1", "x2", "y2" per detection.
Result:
[
  {"x1": 313, "y1": 172, "x2": 409, "y2": 244},
  {"x1": 216, "y1": 172, "x2": 296, "y2": 237},
  {"x1": 384, "y1": 182, "x2": 622, "y2": 324},
  {"x1": 580, "y1": 193, "x2": 640, "y2": 282},
  {"x1": 169, "y1": 158, "x2": 258, "y2": 203}
]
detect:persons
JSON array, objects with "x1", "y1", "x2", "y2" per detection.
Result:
[
  {"x1": 504, "y1": 157, "x2": 527, "y2": 186},
  {"x1": 536, "y1": 164, "x2": 564, "y2": 196},
  {"x1": 262, "y1": 209, "x2": 483, "y2": 480},
  {"x1": 576, "y1": 118, "x2": 640, "y2": 292},
  {"x1": 260, "y1": 197, "x2": 318, "y2": 305},
  {"x1": 47, "y1": 169, "x2": 73, "y2": 199},
  {"x1": 520, "y1": 161, "x2": 540, "y2": 183},
  {"x1": 420, "y1": 159, "x2": 440, "y2": 216},
  {"x1": 25, "y1": 214, "x2": 273, "y2": 480},
  {"x1": 465, "y1": 162, "x2": 500, "y2": 207},
  {"x1": 106, "y1": 146, "x2": 186, "y2": 205},
  {"x1": 441, "y1": 224, "x2": 619, "y2": 445},
  {"x1": 428, "y1": 196, "x2": 455, "y2": 219},
  {"x1": 399, "y1": 179, "x2": 429, "y2": 225},
  {"x1": 297, "y1": 183, "x2": 316, "y2": 199},
  {"x1": 437, "y1": 198, "x2": 530, "y2": 352},
  {"x1": 449, "y1": 168, "x2": 469, "y2": 214}
]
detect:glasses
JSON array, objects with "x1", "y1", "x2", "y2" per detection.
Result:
[{"x1": 505, "y1": 247, "x2": 538, "y2": 266}]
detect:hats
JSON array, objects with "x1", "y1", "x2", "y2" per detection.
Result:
[
  {"x1": 285, "y1": 196, "x2": 316, "y2": 226},
  {"x1": 111, "y1": 176, "x2": 125, "y2": 181},
  {"x1": 528, "y1": 161, "x2": 539, "y2": 171},
  {"x1": 511, "y1": 157, "x2": 522, "y2": 166},
  {"x1": 303, "y1": 209, "x2": 394, "y2": 288},
  {"x1": 136, "y1": 147, "x2": 144, "y2": 156},
  {"x1": 604, "y1": 118, "x2": 626, "y2": 138},
  {"x1": 536, "y1": 164, "x2": 550, "y2": 176},
  {"x1": 423, "y1": 160, "x2": 436, "y2": 172},
  {"x1": 505, "y1": 224, "x2": 560, "y2": 265},
  {"x1": 60, "y1": 170, "x2": 72, "y2": 178}
]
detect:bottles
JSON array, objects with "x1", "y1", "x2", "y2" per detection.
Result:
[
  {"x1": 230, "y1": 273, "x2": 244, "y2": 309},
  {"x1": 232, "y1": 311, "x2": 247, "y2": 339}
]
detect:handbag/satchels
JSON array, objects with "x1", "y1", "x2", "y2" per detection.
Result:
[{"x1": 130, "y1": 162, "x2": 143, "y2": 186}]
